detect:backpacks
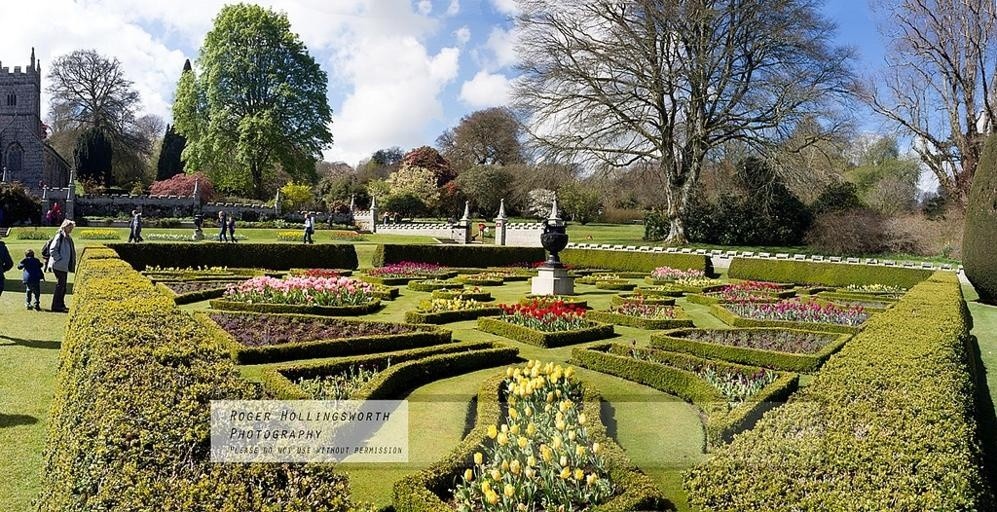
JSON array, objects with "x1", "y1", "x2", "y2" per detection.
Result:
[{"x1": 41, "y1": 234, "x2": 63, "y2": 257}]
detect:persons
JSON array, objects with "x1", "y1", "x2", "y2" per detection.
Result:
[
  {"x1": 133, "y1": 211, "x2": 143, "y2": 243},
  {"x1": 128, "y1": 210, "x2": 140, "y2": 242},
  {"x1": 46, "y1": 218, "x2": 76, "y2": 312},
  {"x1": 0, "y1": 241, "x2": 13, "y2": 295},
  {"x1": 383, "y1": 212, "x2": 389, "y2": 225},
  {"x1": 46, "y1": 202, "x2": 63, "y2": 226},
  {"x1": 217, "y1": 215, "x2": 228, "y2": 242},
  {"x1": 17, "y1": 249, "x2": 45, "y2": 311},
  {"x1": 303, "y1": 214, "x2": 313, "y2": 244},
  {"x1": 393, "y1": 212, "x2": 401, "y2": 225},
  {"x1": 228, "y1": 217, "x2": 237, "y2": 243}
]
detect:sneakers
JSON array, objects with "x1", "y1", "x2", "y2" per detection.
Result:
[
  {"x1": 34, "y1": 305, "x2": 41, "y2": 311},
  {"x1": 26, "y1": 304, "x2": 32, "y2": 309}
]
